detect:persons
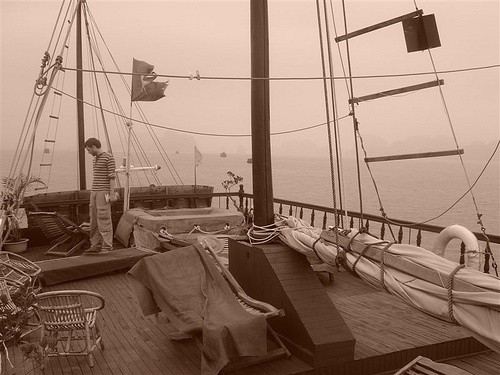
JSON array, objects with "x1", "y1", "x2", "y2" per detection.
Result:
[{"x1": 82, "y1": 138, "x2": 119, "y2": 255}]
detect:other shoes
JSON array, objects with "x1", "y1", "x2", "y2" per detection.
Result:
[
  {"x1": 84, "y1": 248, "x2": 102, "y2": 253},
  {"x1": 100, "y1": 247, "x2": 114, "y2": 254}
]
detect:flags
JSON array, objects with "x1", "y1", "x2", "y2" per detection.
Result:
[{"x1": 131, "y1": 57, "x2": 168, "y2": 102}]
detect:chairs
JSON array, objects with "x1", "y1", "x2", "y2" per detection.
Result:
[
  {"x1": 26, "y1": 290, "x2": 107, "y2": 369},
  {"x1": 27, "y1": 212, "x2": 94, "y2": 258},
  {"x1": 0, "y1": 250, "x2": 43, "y2": 327}
]
